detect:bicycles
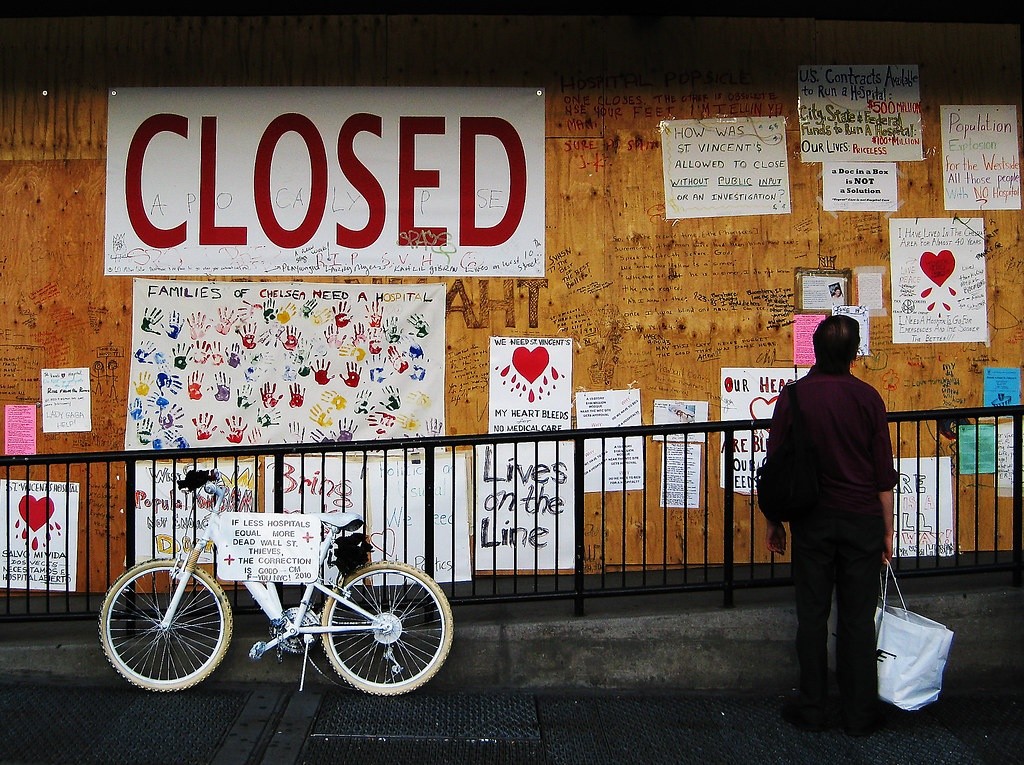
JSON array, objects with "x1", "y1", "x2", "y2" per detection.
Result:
[{"x1": 96, "y1": 467, "x2": 456, "y2": 699}]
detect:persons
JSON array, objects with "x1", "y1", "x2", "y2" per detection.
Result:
[
  {"x1": 757, "y1": 315, "x2": 899, "y2": 737},
  {"x1": 832, "y1": 287, "x2": 843, "y2": 299}
]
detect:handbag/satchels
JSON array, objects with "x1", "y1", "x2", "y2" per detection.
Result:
[
  {"x1": 757, "y1": 381, "x2": 819, "y2": 523},
  {"x1": 827, "y1": 558, "x2": 954, "y2": 711}
]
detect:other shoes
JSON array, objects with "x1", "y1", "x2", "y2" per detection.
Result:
[
  {"x1": 782, "y1": 701, "x2": 825, "y2": 731},
  {"x1": 845, "y1": 713, "x2": 886, "y2": 736}
]
